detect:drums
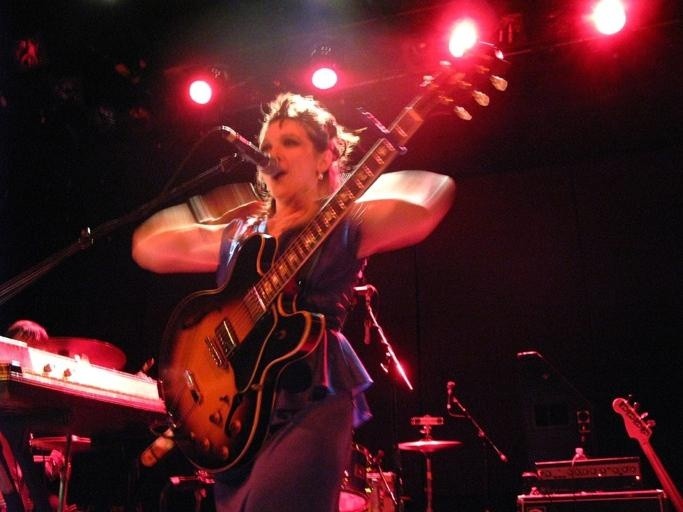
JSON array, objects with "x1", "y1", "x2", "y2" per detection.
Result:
[{"x1": 338, "y1": 443, "x2": 398, "y2": 512}]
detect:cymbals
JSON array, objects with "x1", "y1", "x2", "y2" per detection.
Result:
[
  {"x1": 29, "y1": 333, "x2": 128, "y2": 370},
  {"x1": 397, "y1": 440, "x2": 463, "y2": 451},
  {"x1": 30, "y1": 437, "x2": 92, "y2": 453}
]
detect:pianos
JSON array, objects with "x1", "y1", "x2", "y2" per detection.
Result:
[
  {"x1": 516, "y1": 487, "x2": 665, "y2": 508},
  {"x1": 534, "y1": 457, "x2": 642, "y2": 481},
  {"x1": 0, "y1": 335, "x2": 166, "y2": 421}
]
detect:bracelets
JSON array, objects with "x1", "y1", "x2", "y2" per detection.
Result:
[{"x1": 187, "y1": 194, "x2": 209, "y2": 223}]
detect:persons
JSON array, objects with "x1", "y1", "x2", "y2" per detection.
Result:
[
  {"x1": 130, "y1": 90, "x2": 458, "y2": 511},
  {"x1": 6, "y1": 319, "x2": 51, "y2": 345}
]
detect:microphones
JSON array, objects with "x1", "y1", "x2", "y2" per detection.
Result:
[
  {"x1": 515, "y1": 348, "x2": 545, "y2": 364},
  {"x1": 446, "y1": 381, "x2": 455, "y2": 409},
  {"x1": 221, "y1": 126, "x2": 280, "y2": 176}
]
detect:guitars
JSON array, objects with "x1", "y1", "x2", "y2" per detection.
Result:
[
  {"x1": 612, "y1": 398, "x2": 683, "y2": 509},
  {"x1": 160, "y1": 42, "x2": 514, "y2": 481}
]
